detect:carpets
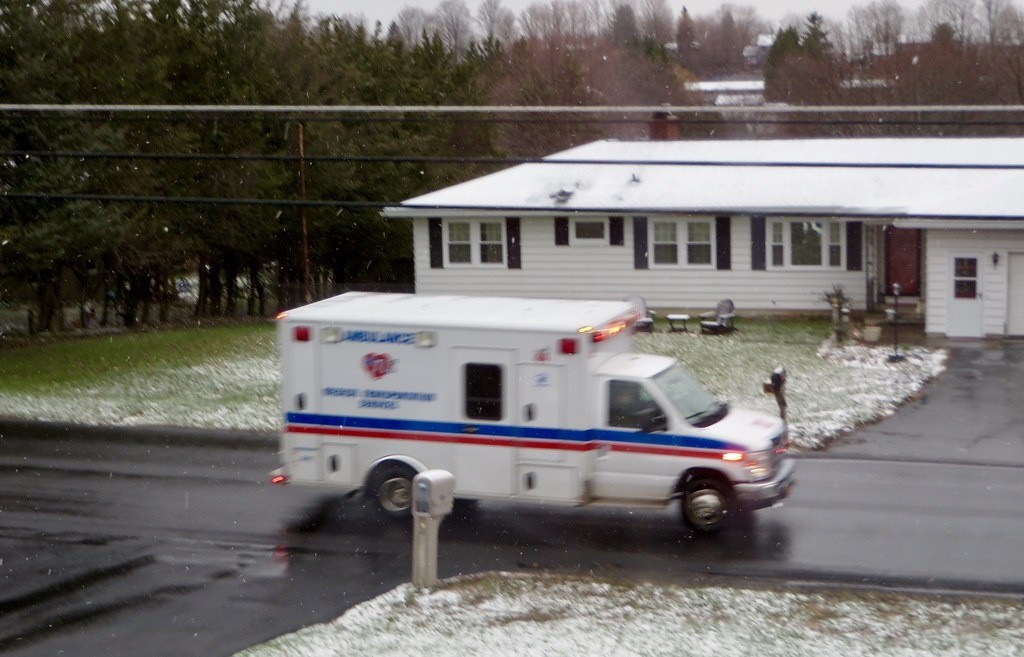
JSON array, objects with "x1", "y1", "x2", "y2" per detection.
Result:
[{"x1": 890, "y1": 302, "x2": 917, "y2": 307}]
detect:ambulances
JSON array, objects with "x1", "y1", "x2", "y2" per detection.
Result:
[{"x1": 268, "y1": 289, "x2": 798, "y2": 539}]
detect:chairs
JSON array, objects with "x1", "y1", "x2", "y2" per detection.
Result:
[
  {"x1": 623, "y1": 295, "x2": 656, "y2": 332},
  {"x1": 698, "y1": 299, "x2": 736, "y2": 333}
]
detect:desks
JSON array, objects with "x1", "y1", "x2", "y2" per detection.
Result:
[{"x1": 666, "y1": 314, "x2": 690, "y2": 331}]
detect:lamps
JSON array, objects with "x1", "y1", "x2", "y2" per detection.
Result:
[{"x1": 992, "y1": 251, "x2": 1000, "y2": 264}]
